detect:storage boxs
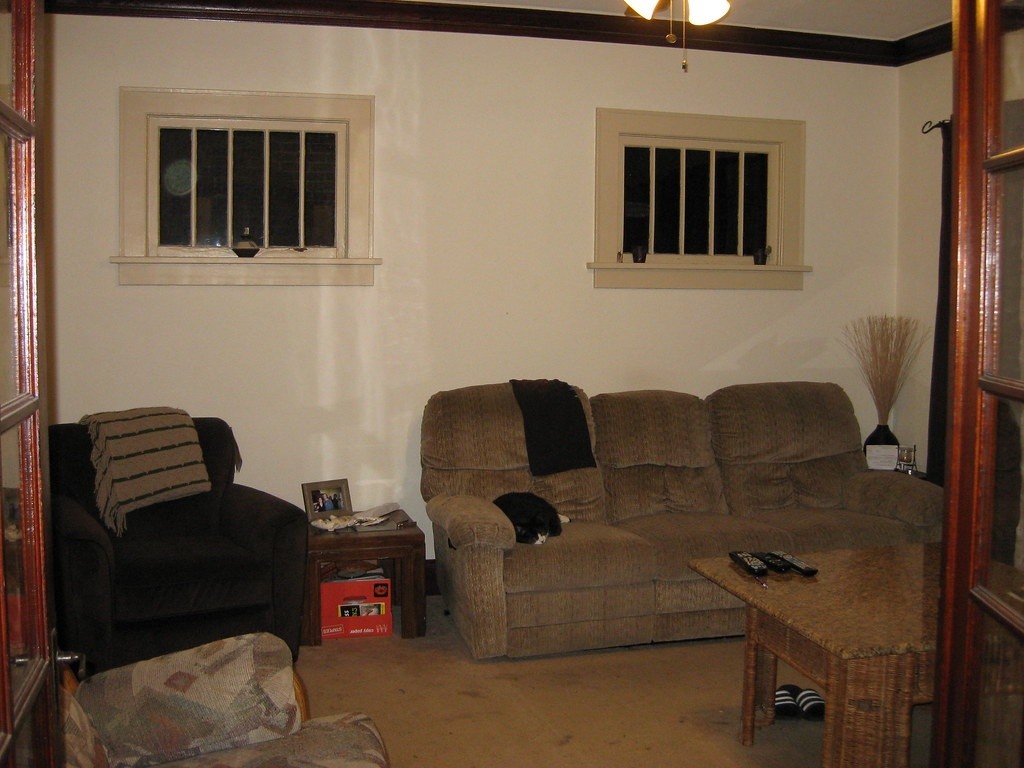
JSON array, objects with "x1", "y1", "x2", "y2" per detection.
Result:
[{"x1": 319, "y1": 573, "x2": 395, "y2": 641}]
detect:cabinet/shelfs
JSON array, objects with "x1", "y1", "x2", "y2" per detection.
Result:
[{"x1": 929, "y1": 0, "x2": 1024, "y2": 768}]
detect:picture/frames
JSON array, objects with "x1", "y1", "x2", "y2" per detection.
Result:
[{"x1": 301, "y1": 477, "x2": 356, "y2": 520}]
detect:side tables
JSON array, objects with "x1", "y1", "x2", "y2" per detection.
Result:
[{"x1": 302, "y1": 508, "x2": 426, "y2": 647}]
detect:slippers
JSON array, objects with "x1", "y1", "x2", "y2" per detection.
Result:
[{"x1": 776, "y1": 683, "x2": 825, "y2": 721}]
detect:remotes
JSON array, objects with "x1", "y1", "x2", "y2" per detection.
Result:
[
  {"x1": 771, "y1": 550, "x2": 818, "y2": 576},
  {"x1": 729, "y1": 551, "x2": 768, "y2": 575},
  {"x1": 751, "y1": 552, "x2": 791, "y2": 573}
]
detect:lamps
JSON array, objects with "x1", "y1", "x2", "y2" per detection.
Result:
[{"x1": 619, "y1": 0, "x2": 736, "y2": 74}]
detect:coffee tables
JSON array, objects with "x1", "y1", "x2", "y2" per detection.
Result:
[{"x1": 684, "y1": 543, "x2": 948, "y2": 768}]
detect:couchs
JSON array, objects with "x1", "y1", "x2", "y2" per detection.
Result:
[
  {"x1": 59, "y1": 629, "x2": 389, "y2": 768},
  {"x1": 45, "y1": 418, "x2": 308, "y2": 681},
  {"x1": 419, "y1": 380, "x2": 946, "y2": 662}
]
